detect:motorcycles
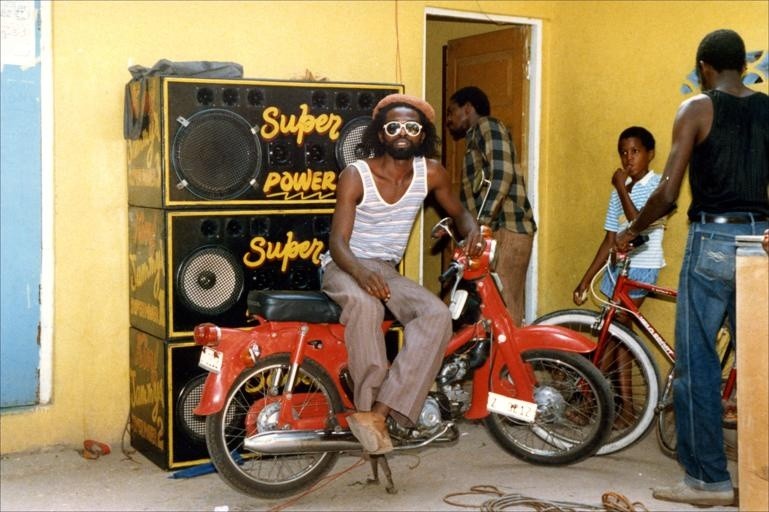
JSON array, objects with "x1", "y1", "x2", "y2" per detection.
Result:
[{"x1": 188, "y1": 166, "x2": 619, "y2": 502}]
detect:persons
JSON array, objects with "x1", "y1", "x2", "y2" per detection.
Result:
[
  {"x1": 614, "y1": 30, "x2": 769, "y2": 506},
  {"x1": 565, "y1": 127, "x2": 667, "y2": 431},
  {"x1": 320, "y1": 92, "x2": 488, "y2": 457},
  {"x1": 446, "y1": 87, "x2": 537, "y2": 326}
]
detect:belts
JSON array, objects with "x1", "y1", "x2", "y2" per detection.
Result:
[{"x1": 689, "y1": 214, "x2": 769, "y2": 224}]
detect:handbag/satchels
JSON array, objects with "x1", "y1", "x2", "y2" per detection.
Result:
[{"x1": 128, "y1": 60, "x2": 243, "y2": 79}]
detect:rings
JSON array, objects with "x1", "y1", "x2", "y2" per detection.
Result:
[{"x1": 477, "y1": 241, "x2": 482, "y2": 247}]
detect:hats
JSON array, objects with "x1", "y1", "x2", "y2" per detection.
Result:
[{"x1": 373, "y1": 93, "x2": 435, "y2": 125}]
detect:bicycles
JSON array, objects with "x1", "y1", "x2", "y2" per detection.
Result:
[{"x1": 513, "y1": 203, "x2": 737, "y2": 459}]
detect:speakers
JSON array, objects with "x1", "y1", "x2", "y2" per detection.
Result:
[
  {"x1": 128, "y1": 206, "x2": 404, "y2": 341},
  {"x1": 128, "y1": 326, "x2": 402, "y2": 470},
  {"x1": 125, "y1": 76, "x2": 404, "y2": 210}
]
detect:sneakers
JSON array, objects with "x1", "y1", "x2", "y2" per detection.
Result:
[
  {"x1": 346, "y1": 412, "x2": 392, "y2": 455},
  {"x1": 653, "y1": 481, "x2": 734, "y2": 506}
]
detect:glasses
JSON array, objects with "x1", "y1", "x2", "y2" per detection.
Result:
[{"x1": 384, "y1": 121, "x2": 423, "y2": 137}]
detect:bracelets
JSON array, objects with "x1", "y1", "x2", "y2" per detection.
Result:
[{"x1": 625, "y1": 226, "x2": 639, "y2": 237}]
